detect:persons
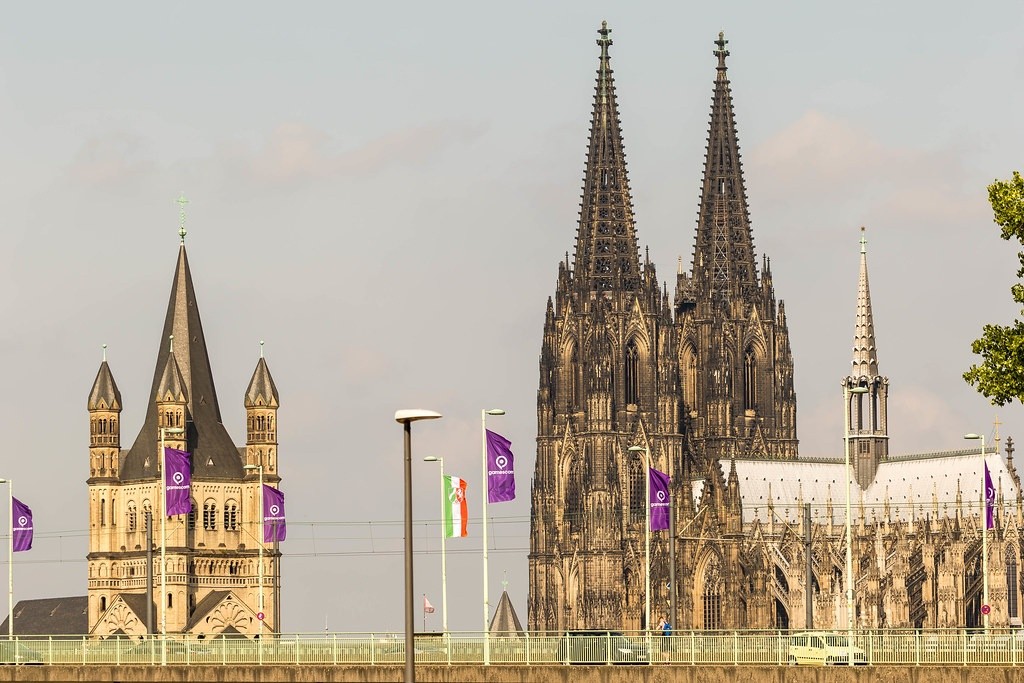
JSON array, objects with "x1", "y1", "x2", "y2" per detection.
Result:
[{"x1": 656, "y1": 618, "x2": 671, "y2": 661}]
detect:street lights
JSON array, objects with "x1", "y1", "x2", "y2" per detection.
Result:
[
  {"x1": 242, "y1": 464, "x2": 264, "y2": 636},
  {"x1": 394, "y1": 409, "x2": 444, "y2": 683},
  {"x1": 0, "y1": 478, "x2": 14, "y2": 642},
  {"x1": 423, "y1": 455, "x2": 448, "y2": 643},
  {"x1": 963, "y1": 432, "x2": 991, "y2": 635},
  {"x1": 844, "y1": 386, "x2": 870, "y2": 667},
  {"x1": 159, "y1": 427, "x2": 184, "y2": 666},
  {"x1": 627, "y1": 444, "x2": 652, "y2": 645},
  {"x1": 481, "y1": 408, "x2": 506, "y2": 666}
]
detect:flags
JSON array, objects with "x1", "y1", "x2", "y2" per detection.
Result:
[
  {"x1": 442, "y1": 474, "x2": 468, "y2": 538},
  {"x1": 485, "y1": 428, "x2": 516, "y2": 503},
  {"x1": 261, "y1": 484, "x2": 286, "y2": 543},
  {"x1": 424, "y1": 598, "x2": 435, "y2": 613},
  {"x1": 647, "y1": 468, "x2": 670, "y2": 531},
  {"x1": 162, "y1": 447, "x2": 192, "y2": 516},
  {"x1": 982, "y1": 460, "x2": 993, "y2": 530},
  {"x1": 11, "y1": 496, "x2": 33, "y2": 552}
]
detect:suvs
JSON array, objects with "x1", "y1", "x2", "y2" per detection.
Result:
[
  {"x1": 788, "y1": 633, "x2": 869, "y2": 665},
  {"x1": 554, "y1": 628, "x2": 650, "y2": 665}
]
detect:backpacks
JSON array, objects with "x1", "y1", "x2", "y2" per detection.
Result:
[{"x1": 662, "y1": 621, "x2": 672, "y2": 636}]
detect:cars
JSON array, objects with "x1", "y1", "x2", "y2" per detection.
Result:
[
  {"x1": 124, "y1": 639, "x2": 211, "y2": 655},
  {"x1": 0, "y1": 641, "x2": 43, "y2": 662},
  {"x1": 385, "y1": 644, "x2": 428, "y2": 655}
]
